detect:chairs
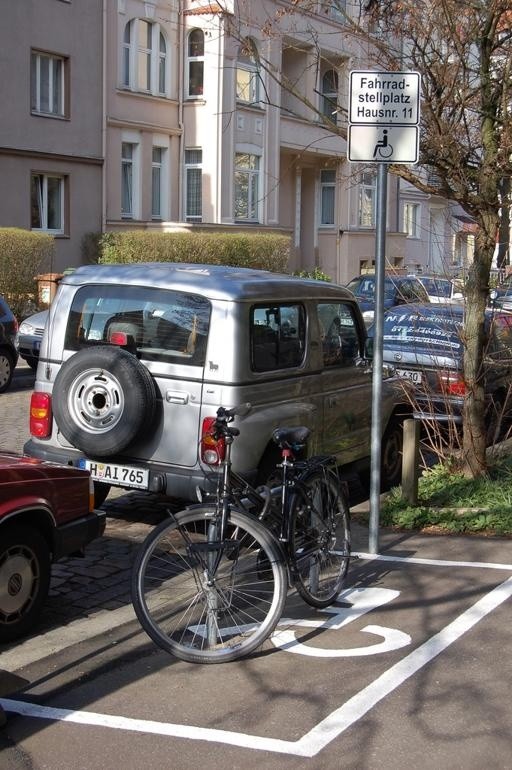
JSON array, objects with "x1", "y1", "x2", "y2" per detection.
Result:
[
  {"x1": 251, "y1": 326, "x2": 277, "y2": 344},
  {"x1": 104, "y1": 314, "x2": 143, "y2": 346}
]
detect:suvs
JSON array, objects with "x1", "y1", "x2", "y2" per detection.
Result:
[{"x1": 25, "y1": 261, "x2": 418, "y2": 505}]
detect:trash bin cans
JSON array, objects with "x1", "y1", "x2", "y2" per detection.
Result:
[{"x1": 33, "y1": 273, "x2": 65, "y2": 307}]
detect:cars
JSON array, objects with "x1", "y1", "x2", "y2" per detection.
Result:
[
  {"x1": 1, "y1": 448, "x2": 108, "y2": 643},
  {"x1": 0, "y1": 295, "x2": 117, "y2": 395},
  {"x1": 344, "y1": 272, "x2": 511, "y2": 449}
]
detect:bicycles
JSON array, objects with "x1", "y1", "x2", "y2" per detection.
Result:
[{"x1": 131, "y1": 404, "x2": 350, "y2": 665}]
